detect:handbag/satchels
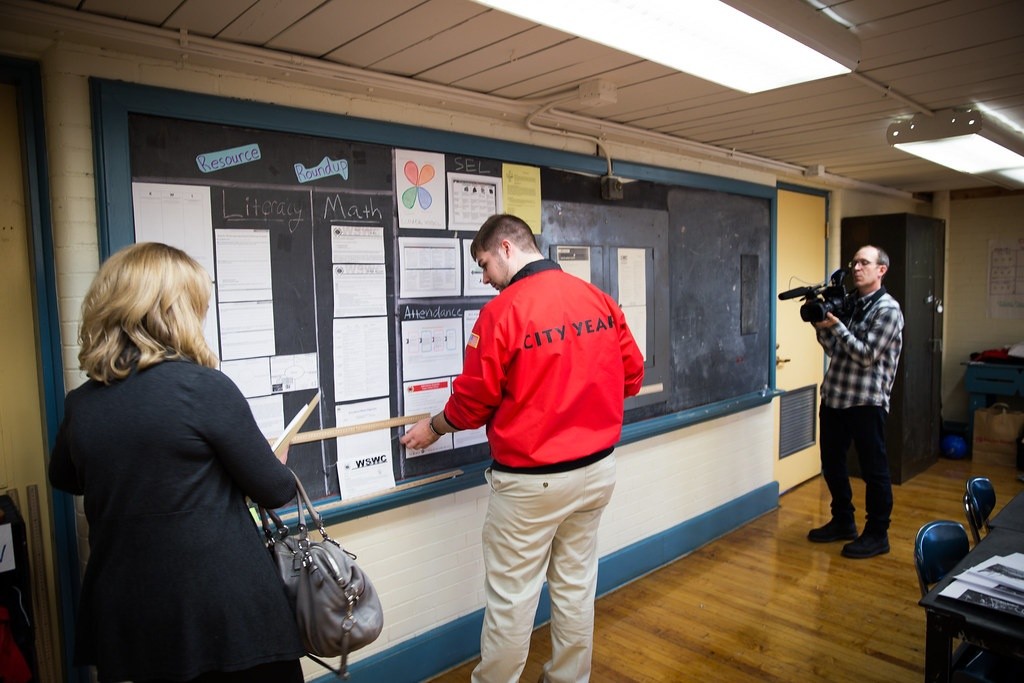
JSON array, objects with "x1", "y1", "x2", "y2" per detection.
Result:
[{"x1": 259, "y1": 468, "x2": 383, "y2": 681}]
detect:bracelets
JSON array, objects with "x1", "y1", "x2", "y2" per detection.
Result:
[{"x1": 429, "y1": 417, "x2": 445, "y2": 436}]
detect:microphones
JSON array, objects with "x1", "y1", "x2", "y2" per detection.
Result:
[{"x1": 778, "y1": 286, "x2": 808, "y2": 300}]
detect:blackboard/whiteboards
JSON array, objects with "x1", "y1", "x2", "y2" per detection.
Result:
[{"x1": 87, "y1": 75, "x2": 778, "y2": 531}]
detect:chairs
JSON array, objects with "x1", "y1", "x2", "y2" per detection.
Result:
[
  {"x1": 963, "y1": 476, "x2": 997, "y2": 550},
  {"x1": 914, "y1": 519, "x2": 970, "y2": 595}
]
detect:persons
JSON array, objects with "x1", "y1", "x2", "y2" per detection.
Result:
[
  {"x1": 397, "y1": 212, "x2": 644, "y2": 683},
  {"x1": 49, "y1": 241, "x2": 306, "y2": 683},
  {"x1": 806, "y1": 245, "x2": 904, "y2": 559}
]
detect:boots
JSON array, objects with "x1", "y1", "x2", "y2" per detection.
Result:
[
  {"x1": 841, "y1": 515, "x2": 891, "y2": 558},
  {"x1": 809, "y1": 511, "x2": 858, "y2": 542}
]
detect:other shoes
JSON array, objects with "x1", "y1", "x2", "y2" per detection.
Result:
[{"x1": 538, "y1": 673, "x2": 546, "y2": 683}]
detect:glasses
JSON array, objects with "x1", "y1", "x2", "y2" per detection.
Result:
[{"x1": 848, "y1": 261, "x2": 877, "y2": 268}]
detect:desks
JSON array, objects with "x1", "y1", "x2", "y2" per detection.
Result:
[
  {"x1": 917, "y1": 487, "x2": 1024, "y2": 683},
  {"x1": 964, "y1": 363, "x2": 1024, "y2": 453}
]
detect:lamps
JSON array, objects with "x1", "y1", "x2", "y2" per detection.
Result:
[
  {"x1": 472, "y1": 0, "x2": 864, "y2": 97},
  {"x1": 885, "y1": 108, "x2": 1024, "y2": 190}
]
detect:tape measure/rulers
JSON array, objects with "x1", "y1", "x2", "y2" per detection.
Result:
[
  {"x1": 257, "y1": 468, "x2": 464, "y2": 528},
  {"x1": 636, "y1": 382, "x2": 663, "y2": 397},
  {"x1": 267, "y1": 410, "x2": 431, "y2": 451}
]
